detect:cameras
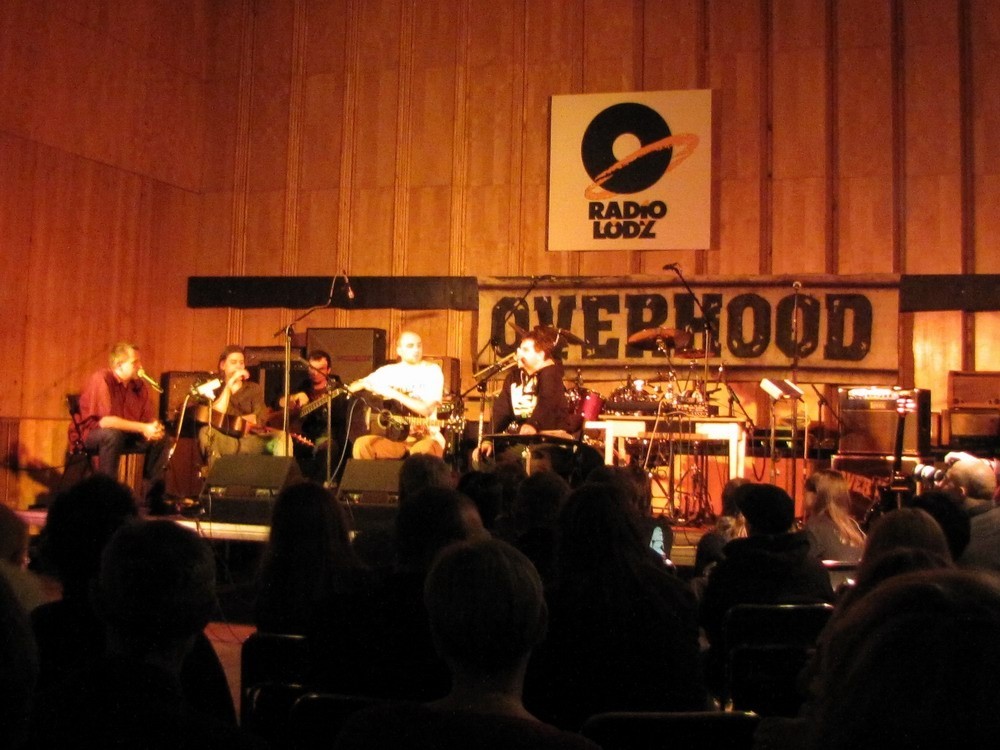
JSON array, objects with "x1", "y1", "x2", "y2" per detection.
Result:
[{"x1": 913, "y1": 464, "x2": 945, "y2": 488}]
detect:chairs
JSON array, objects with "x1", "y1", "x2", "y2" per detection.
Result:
[
  {"x1": 588, "y1": 707, "x2": 760, "y2": 750},
  {"x1": 704, "y1": 596, "x2": 833, "y2": 706},
  {"x1": 242, "y1": 627, "x2": 337, "y2": 731}
]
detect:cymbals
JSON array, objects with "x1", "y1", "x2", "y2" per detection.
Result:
[
  {"x1": 626, "y1": 328, "x2": 690, "y2": 352},
  {"x1": 674, "y1": 349, "x2": 715, "y2": 360},
  {"x1": 533, "y1": 325, "x2": 585, "y2": 347},
  {"x1": 509, "y1": 322, "x2": 526, "y2": 338}
]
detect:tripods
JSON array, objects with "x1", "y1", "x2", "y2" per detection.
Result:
[{"x1": 659, "y1": 271, "x2": 721, "y2": 532}]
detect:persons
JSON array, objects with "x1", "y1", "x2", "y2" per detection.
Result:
[
  {"x1": 280, "y1": 349, "x2": 354, "y2": 480},
  {"x1": 464, "y1": 324, "x2": 576, "y2": 474},
  {"x1": 0, "y1": 446, "x2": 999, "y2": 750},
  {"x1": 344, "y1": 330, "x2": 450, "y2": 461},
  {"x1": 63, "y1": 339, "x2": 178, "y2": 515},
  {"x1": 197, "y1": 341, "x2": 266, "y2": 463}
]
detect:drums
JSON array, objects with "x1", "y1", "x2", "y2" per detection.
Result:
[
  {"x1": 610, "y1": 387, "x2": 651, "y2": 416},
  {"x1": 566, "y1": 387, "x2": 604, "y2": 422}
]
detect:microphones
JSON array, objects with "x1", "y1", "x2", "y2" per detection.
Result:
[
  {"x1": 532, "y1": 275, "x2": 557, "y2": 283},
  {"x1": 343, "y1": 269, "x2": 354, "y2": 299},
  {"x1": 137, "y1": 369, "x2": 162, "y2": 389},
  {"x1": 663, "y1": 263, "x2": 679, "y2": 270}
]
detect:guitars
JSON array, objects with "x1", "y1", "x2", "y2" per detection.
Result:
[
  {"x1": 184, "y1": 403, "x2": 314, "y2": 449},
  {"x1": 346, "y1": 395, "x2": 466, "y2": 442},
  {"x1": 258, "y1": 384, "x2": 347, "y2": 433}
]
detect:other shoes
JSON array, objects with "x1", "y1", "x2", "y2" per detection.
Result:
[{"x1": 148, "y1": 499, "x2": 179, "y2": 515}]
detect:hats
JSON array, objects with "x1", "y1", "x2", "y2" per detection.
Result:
[{"x1": 736, "y1": 481, "x2": 795, "y2": 532}]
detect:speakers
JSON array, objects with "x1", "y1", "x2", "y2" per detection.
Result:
[
  {"x1": 336, "y1": 459, "x2": 453, "y2": 531},
  {"x1": 204, "y1": 454, "x2": 303, "y2": 518},
  {"x1": 947, "y1": 369, "x2": 1000, "y2": 450},
  {"x1": 836, "y1": 386, "x2": 930, "y2": 456}
]
